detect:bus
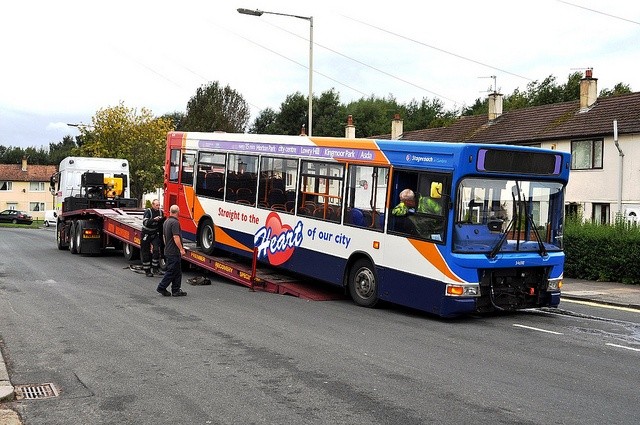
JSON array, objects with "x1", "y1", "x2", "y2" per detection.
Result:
[{"x1": 162, "y1": 129, "x2": 572, "y2": 321}]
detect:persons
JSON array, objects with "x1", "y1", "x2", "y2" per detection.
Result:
[
  {"x1": 141, "y1": 199, "x2": 167, "y2": 277},
  {"x1": 392, "y1": 189, "x2": 443, "y2": 238},
  {"x1": 156, "y1": 205, "x2": 187, "y2": 296},
  {"x1": 355, "y1": 180, "x2": 372, "y2": 208}
]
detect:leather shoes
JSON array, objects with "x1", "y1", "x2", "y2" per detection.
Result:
[
  {"x1": 152, "y1": 268, "x2": 164, "y2": 276},
  {"x1": 143, "y1": 270, "x2": 154, "y2": 277}
]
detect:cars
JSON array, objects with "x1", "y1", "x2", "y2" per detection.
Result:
[
  {"x1": 0, "y1": 210, "x2": 33, "y2": 225},
  {"x1": 44, "y1": 210, "x2": 55, "y2": 227}
]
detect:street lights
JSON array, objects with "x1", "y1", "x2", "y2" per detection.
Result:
[
  {"x1": 68, "y1": 123, "x2": 94, "y2": 128},
  {"x1": 237, "y1": 9, "x2": 315, "y2": 140}
]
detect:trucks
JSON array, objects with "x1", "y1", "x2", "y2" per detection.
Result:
[{"x1": 47, "y1": 155, "x2": 345, "y2": 300}]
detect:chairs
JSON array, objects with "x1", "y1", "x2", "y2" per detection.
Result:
[
  {"x1": 218, "y1": 187, "x2": 234, "y2": 202},
  {"x1": 350, "y1": 209, "x2": 369, "y2": 227},
  {"x1": 364, "y1": 210, "x2": 371, "y2": 225},
  {"x1": 270, "y1": 178, "x2": 284, "y2": 190},
  {"x1": 257, "y1": 201, "x2": 269, "y2": 209},
  {"x1": 267, "y1": 188, "x2": 285, "y2": 209},
  {"x1": 305, "y1": 200, "x2": 317, "y2": 206},
  {"x1": 237, "y1": 199, "x2": 251, "y2": 207},
  {"x1": 336, "y1": 208, "x2": 341, "y2": 219},
  {"x1": 284, "y1": 201, "x2": 294, "y2": 214},
  {"x1": 178, "y1": 170, "x2": 192, "y2": 184},
  {"x1": 236, "y1": 188, "x2": 254, "y2": 203},
  {"x1": 380, "y1": 213, "x2": 384, "y2": 230},
  {"x1": 203, "y1": 171, "x2": 218, "y2": 192},
  {"x1": 312, "y1": 206, "x2": 337, "y2": 223},
  {"x1": 259, "y1": 178, "x2": 268, "y2": 200},
  {"x1": 213, "y1": 174, "x2": 226, "y2": 188},
  {"x1": 286, "y1": 189, "x2": 295, "y2": 201},
  {"x1": 297, "y1": 206, "x2": 311, "y2": 217},
  {"x1": 226, "y1": 173, "x2": 256, "y2": 189},
  {"x1": 304, "y1": 203, "x2": 315, "y2": 212}
]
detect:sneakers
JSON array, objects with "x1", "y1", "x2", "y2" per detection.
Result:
[
  {"x1": 156, "y1": 287, "x2": 171, "y2": 296},
  {"x1": 173, "y1": 289, "x2": 188, "y2": 296}
]
